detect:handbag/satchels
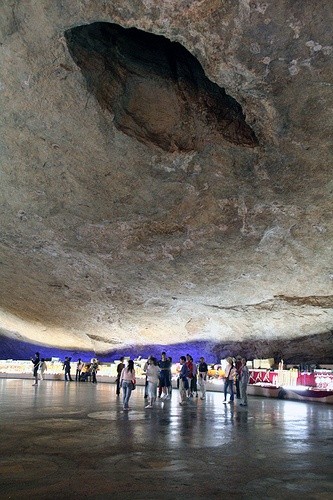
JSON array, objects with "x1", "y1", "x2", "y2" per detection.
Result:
[
  {"x1": 127, "y1": 383, "x2": 135, "y2": 390},
  {"x1": 225, "y1": 377, "x2": 229, "y2": 384}
]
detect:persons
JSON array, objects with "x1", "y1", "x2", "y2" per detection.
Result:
[
  {"x1": 143, "y1": 355, "x2": 153, "y2": 398},
  {"x1": 157, "y1": 352, "x2": 171, "y2": 399},
  {"x1": 222, "y1": 357, "x2": 238, "y2": 403},
  {"x1": 240, "y1": 356, "x2": 250, "y2": 407},
  {"x1": 119, "y1": 360, "x2": 136, "y2": 410},
  {"x1": 234, "y1": 355, "x2": 243, "y2": 399},
  {"x1": 31, "y1": 353, "x2": 39, "y2": 386},
  {"x1": 186, "y1": 353, "x2": 198, "y2": 398},
  {"x1": 115, "y1": 357, "x2": 125, "y2": 397},
  {"x1": 176, "y1": 356, "x2": 190, "y2": 406},
  {"x1": 62, "y1": 356, "x2": 75, "y2": 381},
  {"x1": 39, "y1": 358, "x2": 48, "y2": 380},
  {"x1": 75, "y1": 357, "x2": 99, "y2": 383},
  {"x1": 141, "y1": 358, "x2": 161, "y2": 408},
  {"x1": 198, "y1": 356, "x2": 208, "y2": 400}
]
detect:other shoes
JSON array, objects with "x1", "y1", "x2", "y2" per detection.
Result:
[
  {"x1": 179, "y1": 400, "x2": 188, "y2": 405},
  {"x1": 165, "y1": 395, "x2": 170, "y2": 400},
  {"x1": 200, "y1": 396, "x2": 206, "y2": 400},
  {"x1": 223, "y1": 400, "x2": 226, "y2": 403},
  {"x1": 144, "y1": 395, "x2": 148, "y2": 398},
  {"x1": 189, "y1": 394, "x2": 193, "y2": 397},
  {"x1": 228, "y1": 401, "x2": 233, "y2": 403},
  {"x1": 158, "y1": 395, "x2": 160, "y2": 398},
  {"x1": 196, "y1": 394, "x2": 198, "y2": 397},
  {"x1": 32, "y1": 384, "x2": 38, "y2": 386},
  {"x1": 145, "y1": 405, "x2": 153, "y2": 408},
  {"x1": 161, "y1": 394, "x2": 166, "y2": 399},
  {"x1": 240, "y1": 403, "x2": 248, "y2": 406},
  {"x1": 123, "y1": 407, "x2": 132, "y2": 410}
]
